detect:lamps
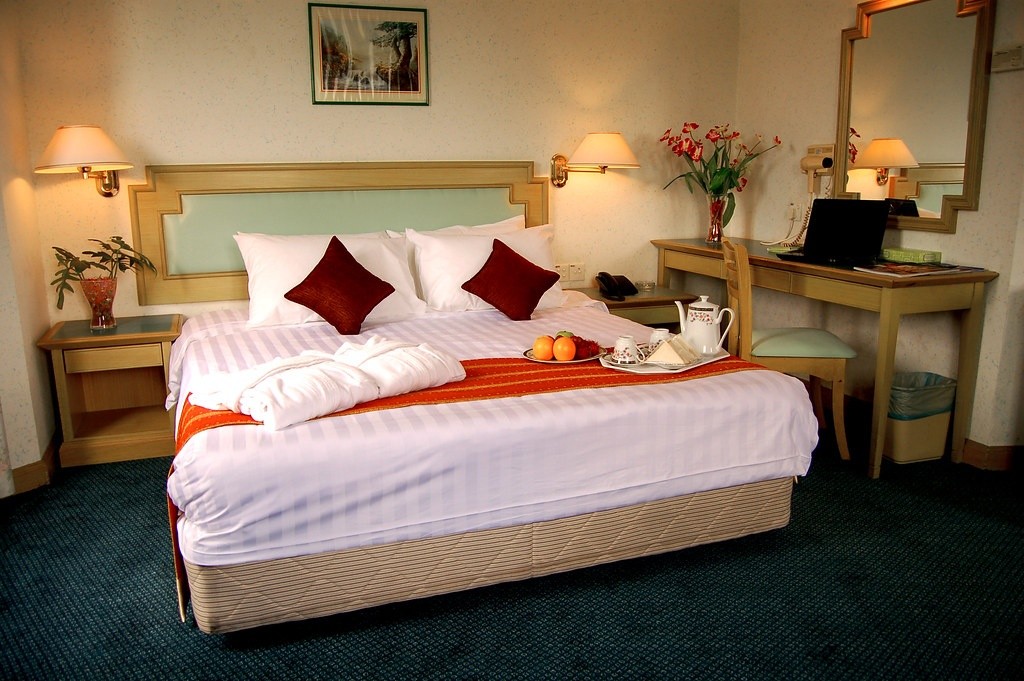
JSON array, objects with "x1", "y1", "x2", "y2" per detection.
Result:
[
  {"x1": 852, "y1": 139, "x2": 919, "y2": 185},
  {"x1": 550, "y1": 134, "x2": 639, "y2": 186},
  {"x1": 32, "y1": 123, "x2": 137, "y2": 197}
]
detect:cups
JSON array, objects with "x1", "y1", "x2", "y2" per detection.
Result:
[
  {"x1": 649, "y1": 328, "x2": 670, "y2": 355},
  {"x1": 611, "y1": 335, "x2": 646, "y2": 363}
]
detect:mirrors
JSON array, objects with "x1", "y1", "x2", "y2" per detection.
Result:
[{"x1": 832, "y1": 0, "x2": 998, "y2": 232}]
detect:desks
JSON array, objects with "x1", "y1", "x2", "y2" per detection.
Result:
[{"x1": 652, "y1": 234, "x2": 998, "y2": 478}]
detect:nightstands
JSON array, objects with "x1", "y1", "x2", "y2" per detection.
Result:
[
  {"x1": 564, "y1": 288, "x2": 697, "y2": 330},
  {"x1": 34, "y1": 313, "x2": 186, "y2": 467}
]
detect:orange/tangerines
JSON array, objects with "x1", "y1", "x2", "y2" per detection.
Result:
[{"x1": 533, "y1": 335, "x2": 575, "y2": 361}]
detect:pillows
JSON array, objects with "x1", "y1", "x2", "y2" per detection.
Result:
[
  {"x1": 403, "y1": 227, "x2": 566, "y2": 313},
  {"x1": 231, "y1": 233, "x2": 412, "y2": 324},
  {"x1": 282, "y1": 235, "x2": 395, "y2": 335},
  {"x1": 458, "y1": 237, "x2": 558, "y2": 320},
  {"x1": 385, "y1": 213, "x2": 522, "y2": 302}
]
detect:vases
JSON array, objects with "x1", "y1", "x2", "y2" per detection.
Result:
[{"x1": 704, "y1": 192, "x2": 728, "y2": 245}]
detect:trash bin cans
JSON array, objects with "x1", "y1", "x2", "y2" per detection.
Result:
[{"x1": 882, "y1": 372, "x2": 958, "y2": 465}]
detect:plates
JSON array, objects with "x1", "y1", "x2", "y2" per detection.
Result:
[
  {"x1": 603, "y1": 354, "x2": 643, "y2": 367},
  {"x1": 523, "y1": 348, "x2": 609, "y2": 363}
]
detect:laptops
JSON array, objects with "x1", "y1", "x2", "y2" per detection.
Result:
[
  {"x1": 885, "y1": 197, "x2": 919, "y2": 218},
  {"x1": 776, "y1": 198, "x2": 895, "y2": 270}
]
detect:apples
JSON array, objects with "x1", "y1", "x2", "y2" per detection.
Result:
[{"x1": 556, "y1": 331, "x2": 574, "y2": 338}]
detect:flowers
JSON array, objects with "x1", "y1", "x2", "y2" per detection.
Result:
[
  {"x1": 658, "y1": 123, "x2": 780, "y2": 227},
  {"x1": 846, "y1": 127, "x2": 861, "y2": 164}
]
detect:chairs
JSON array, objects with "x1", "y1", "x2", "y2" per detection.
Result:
[{"x1": 718, "y1": 235, "x2": 854, "y2": 459}]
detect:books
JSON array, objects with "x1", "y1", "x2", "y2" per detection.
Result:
[{"x1": 853, "y1": 263, "x2": 987, "y2": 278}]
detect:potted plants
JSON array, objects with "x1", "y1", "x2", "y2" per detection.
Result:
[{"x1": 49, "y1": 234, "x2": 158, "y2": 331}]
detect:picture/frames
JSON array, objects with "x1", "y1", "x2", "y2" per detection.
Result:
[{"x1": 307, "y1": 0, "x2": 430, "y2": 106}]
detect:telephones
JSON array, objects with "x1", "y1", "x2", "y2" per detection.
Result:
[{"x1": 595, "y1": 272, "x2": 639, "y2": 296}]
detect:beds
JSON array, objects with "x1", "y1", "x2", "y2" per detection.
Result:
[{"x1": 130, "y1": 161, "x2": 819, "y2": 637}]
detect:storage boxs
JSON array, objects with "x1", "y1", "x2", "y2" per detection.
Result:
[{"x1": 880, "y1": 246, "x2": 943, "y2": 263}]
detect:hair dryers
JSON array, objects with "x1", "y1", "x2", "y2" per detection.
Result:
[{"x1": 799, "y1": 156, "x2": 833, "y2": 192}]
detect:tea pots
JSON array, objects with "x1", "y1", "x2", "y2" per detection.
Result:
[{"x1": 673, "y1": 295, "x2": 736, "y2": 358}]
